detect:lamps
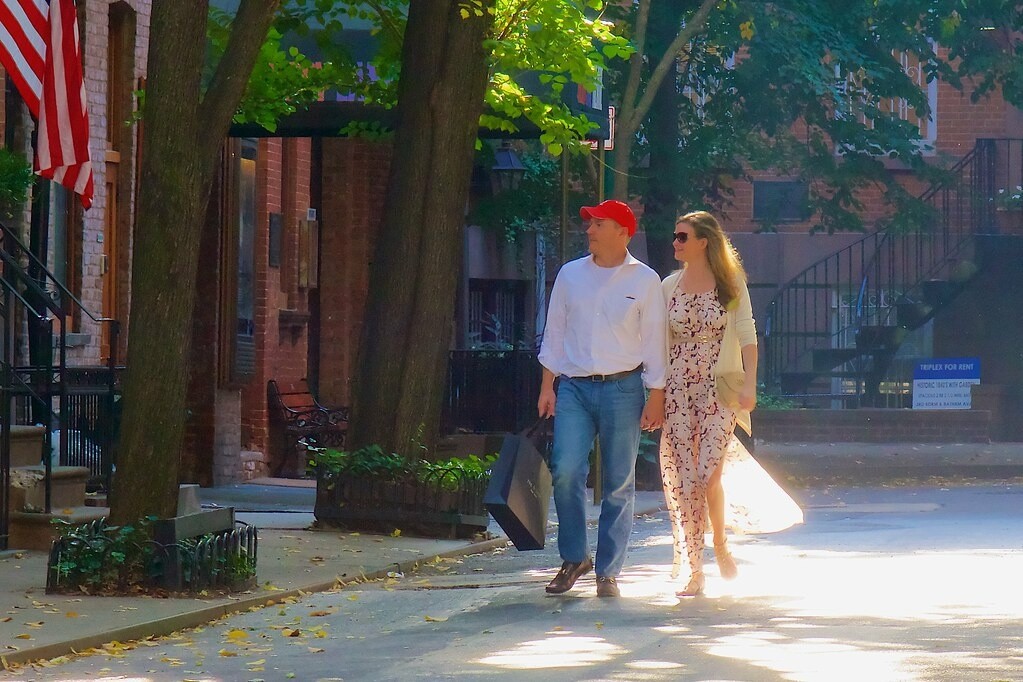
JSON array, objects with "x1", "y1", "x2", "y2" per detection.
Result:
[{"x1": 485, "y1": 139, "x2": 528, "y2": 199}]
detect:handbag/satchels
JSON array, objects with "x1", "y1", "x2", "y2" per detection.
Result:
[{"x1": 482, "y1": 413, "x2": 553, "y2": 551}]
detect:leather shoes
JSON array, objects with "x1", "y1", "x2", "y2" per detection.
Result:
[
  {"x1": 594, "y1": 574, "x2": 619, "y2": 597},
  {"x1": 545, "y1": 553, "x2": 593, "y2": 597}
]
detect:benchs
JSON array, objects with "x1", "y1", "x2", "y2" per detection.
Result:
[{"x1": 268, "y1": 377, "x2": 352, "y2": 475}]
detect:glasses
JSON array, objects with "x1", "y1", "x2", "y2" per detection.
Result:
[{"x1": 672, "y1": 232, "x2": 689, "y2": 244}]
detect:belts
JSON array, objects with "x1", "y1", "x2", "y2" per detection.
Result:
[{"x1": 590, "y1": 361, "x2": 642, "y2": 383}]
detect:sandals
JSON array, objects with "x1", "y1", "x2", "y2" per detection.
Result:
[
  {"x1": 712, "y1": 537, "x2": 737, "y2": 580},
  {"x1": 675, "y1": 570, "x2": 705, "y2": 596}
]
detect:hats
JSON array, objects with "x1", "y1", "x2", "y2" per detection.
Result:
[{"x1": 579, "y1": 200, "x2": 637, "y2": 238}]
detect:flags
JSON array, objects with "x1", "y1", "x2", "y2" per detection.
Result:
[{"x1": 0, "y1": 0, "x2": 94, "y2": 213}]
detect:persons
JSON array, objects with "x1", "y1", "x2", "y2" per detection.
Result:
[
  {"x1": 641, "y1": 211, "x2": 804, "y2": 596},
  {"x1": 537, "y1": 199, "x2": 666, "y2": 597}
]
